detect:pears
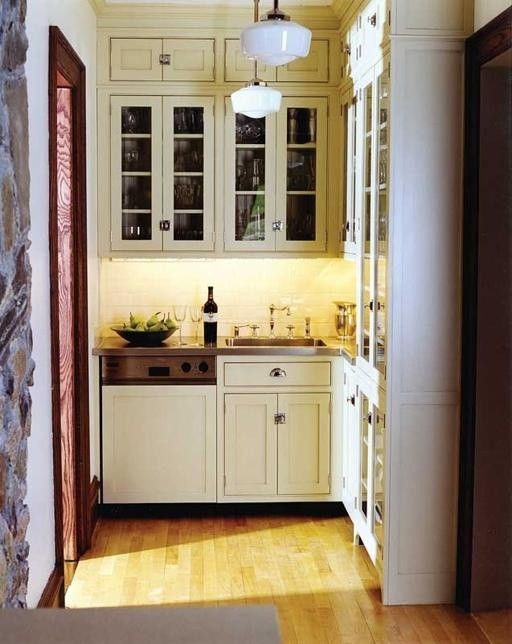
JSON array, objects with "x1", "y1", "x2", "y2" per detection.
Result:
[{"x1": 121, "y1": 311, "x2": 176, "y2": 331}]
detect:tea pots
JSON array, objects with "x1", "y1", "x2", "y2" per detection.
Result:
[{"x1": 332, "y1": 300, "x2": 356, "y2": 339}]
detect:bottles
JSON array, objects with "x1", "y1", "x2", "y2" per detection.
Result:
[
  {"x1": 244, "y1": 158, "x2": 264, "y2": 190},
  {"x1": 202, "y1": 285, "x2": 218, "y2": 346},
  {"x1": 308, "y1": 109, "x2": 316, "y2": 145},
  {"x1": 289, "y1": 109, "x2": 298, "y2": 143},
  {"x1": 175, "y1": 108, "x2": 202, "y2": 134}
]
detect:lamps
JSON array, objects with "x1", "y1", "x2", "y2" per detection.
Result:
[
  {"x1": 228, "y1": 0, "x2": 283, "y2": 121},
  {"x1": 238, "y1": 0, "x2": 314, "y2": 69}
]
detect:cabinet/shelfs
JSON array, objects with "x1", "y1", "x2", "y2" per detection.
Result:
[
  {"x1": 215, "y1": 352, "x2": 340, "y2": 504},
  {"x1": 337, "y1": 32, "x2": 474, "y2": 391},
  {"x1": 221, "y1": 19, "x2": 344, "y2": 262},
  {"x1": 338, "y1": 360, "x2": 463, "y2": 608},
  {"x1": 338, "y1": 0, "x2": 471, "y2": 105},
  {"x1": 96, "y1": 24, "x2": 218, "y2": 262}
]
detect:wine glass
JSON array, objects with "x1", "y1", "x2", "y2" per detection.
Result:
[
  {"x1": 186, "y1": 304, "x2": 204, "y2": 345},
  {"x1": 172, "y1": 303, "x2": 189, "y2": 345}
]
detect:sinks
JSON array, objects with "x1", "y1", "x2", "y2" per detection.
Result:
[{"x1": 225, "y1": 339, "x2": 327, "y2": 346}]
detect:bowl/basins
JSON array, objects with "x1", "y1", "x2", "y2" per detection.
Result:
[{"x1": 111, "y1": 324, "x2": 180, "y2": 345}]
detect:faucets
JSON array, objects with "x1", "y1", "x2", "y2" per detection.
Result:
[{"x1": 268, "y1": 304, "x2": 293, "y2": 338}]
